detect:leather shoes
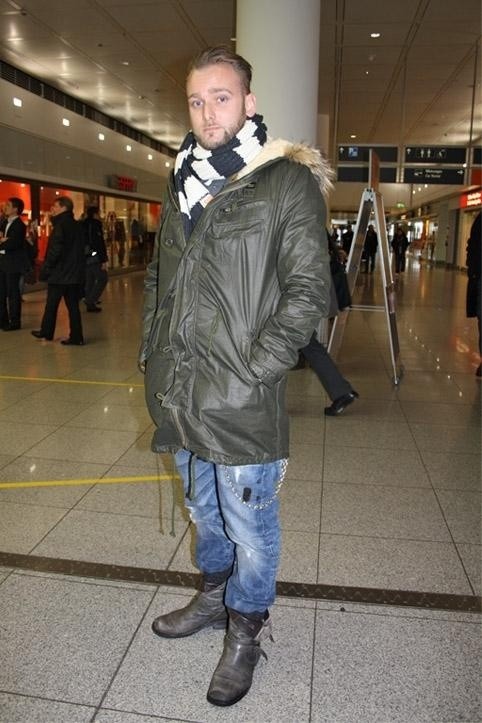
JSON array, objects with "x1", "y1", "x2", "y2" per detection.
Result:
[
  {"x1": 30, "y1": 330, "x2": 53, "y2": 343},
  {"x1": 151, "y1": 559, "x2": 236, "y2": 639},
  {"x1": 206, "y1": 605, "x2": 275, "y2": 709},
  {"x1": 323, "y1": 390, "x2": 360, "y2": 418},
  {"x1": 60, "y1": 338, "x2": 83, "y2": 348}
]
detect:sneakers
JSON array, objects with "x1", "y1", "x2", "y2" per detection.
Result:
[{"x1": 83, "y1": 298, "x2": 102, "y2": 314}]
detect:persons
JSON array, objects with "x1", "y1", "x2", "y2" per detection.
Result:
[
  {"x1": 299, "y1": 222, "x2": 380, "y2": 414},
  {"x1": 465, "y1": 215, "x2": 482, "y2": 377},
  {"x1": 2, "y1": 196, "x2": 146, "y2": 347},
  {"x1": 138, "y1": 42, "x2": 339, "y2": 711},
  {"x1": 392, "y1": 227, "x2": 409, "y2": 274}
]
